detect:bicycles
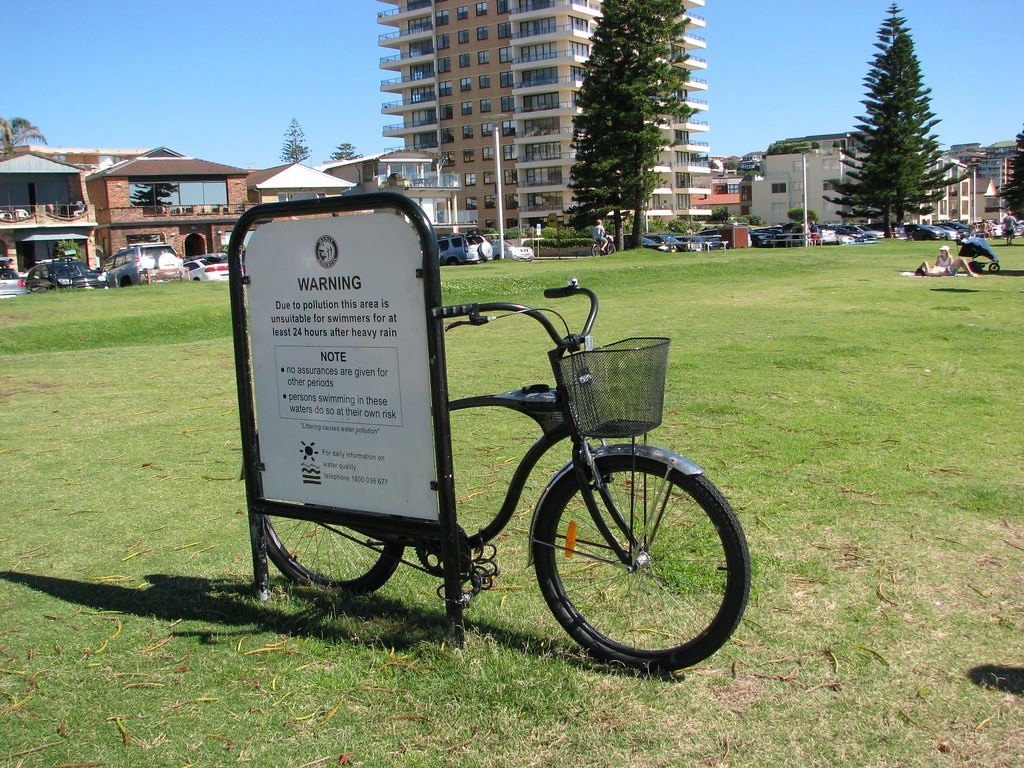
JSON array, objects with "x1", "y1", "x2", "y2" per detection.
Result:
[
  {"x1": 592, "y1": 236, "x2": 616, "y2": 257},
  {"x1": 238, "y1": 279, "x2": 753, "y2": 673}
]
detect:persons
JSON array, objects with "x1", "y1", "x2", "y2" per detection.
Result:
[
  {"x1": 969, "y1": 219, "x2": 993, "y2": 240},
  {"x1": 594, "y1": 219, "x2": 608, "y2": 253},
  {"x1": 915, "y1": 257, "x2": 976, "y2": 277},
  {"x1": 935, "y1": 246, "x2": 953, "y2": 266},
  {"x1": 1002, "y1": 211, "x2": 1019, "y2": 244},
  {"x1": 808, "y1": 220, "x2": 822, "y2": 246}
]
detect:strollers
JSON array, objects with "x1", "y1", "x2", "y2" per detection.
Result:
[{"x1": 957, "y1": 236, "x2": 1001, "y2": 274}]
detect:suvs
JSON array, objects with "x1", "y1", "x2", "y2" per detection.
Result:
[
  {"x1": 437, "y1": 234, "x2": 493, "y2": 265},
  {"x1": 96, "y1": 242, "x2": 185, "y2": 288},
  {"x1": 644, "y1": 234, "x2": 704, "y2": 252},
  {"x1": 867, "y1": 222, "x2": 908, "y2": 240}
]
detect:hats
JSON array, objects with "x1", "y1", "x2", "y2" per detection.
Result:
[{"x1": 939, "y1": 245, "x2": 949, "y2": 252}]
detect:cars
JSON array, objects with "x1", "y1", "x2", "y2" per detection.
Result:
[
  {"x1": 676, "y1": 236, "x2": 721, "y2": 249},
  {"x1": 757, "y1": 222, "x2": 885, "y2": 248},
  {"x1": 185, "y1": 259, "x2": 229, "y2": 282},
  {"x1": 614, "y1": 235, "x2": 679, "y2": 254},
  {"x1": 698, "y1": 226, "x2": 777, "y2": 247},
  {"x1": 928, "y1": 220, "x2": 1024, "y2": 240},
  {"x1": 490, "y1": 240, "x2": 534, "y2": 264},
  {"x1": 26, "y1": 259, "x2": 110, "y2": 293},
  {"x1": 0, "y1": 267, "x2": 27, "y2": 299},
  {"x1": 905, "y1": 224, "x2": 945, "y2": 241}
]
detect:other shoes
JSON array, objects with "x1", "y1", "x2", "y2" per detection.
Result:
[
  {"x1": 967, "y1": 273, "x2": 977, "y2": 276},
  {"x1": 601, "y1": 249, "x2": 605, "y2": 252}
]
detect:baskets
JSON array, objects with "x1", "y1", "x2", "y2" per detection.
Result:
[
  {"x1": 607, "y1": 237, "x2": 613, "y2": 242},
  {"x1": 556, "y1": 336, "x2": 671, "y2": 438}
]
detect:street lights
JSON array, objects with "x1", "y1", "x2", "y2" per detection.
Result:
[
  {"x1": 797, "y1": 146, "x2": 812, "y2": 247},
  {"x1": 997, "y1": 154, "x2": 1018, "y2": 221},
  {"x1": 483, "y1": 113, "x2": 510, "y2": 259},
  {"x1": 967, "y1": 163, "x2": 980, "y2": 225}
]
